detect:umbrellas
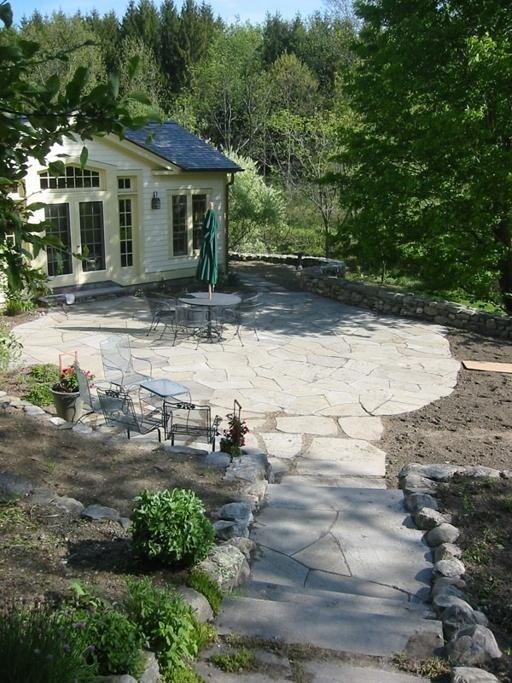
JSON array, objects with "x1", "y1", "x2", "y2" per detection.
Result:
[{"x1": 195, "y1": 202, "x2": 218, "y2": 332}]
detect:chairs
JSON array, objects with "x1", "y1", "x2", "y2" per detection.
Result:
[
  {"x1": 73, "y1": 332, "x2": 222, "y2": 451},
  {"x1": 143, "y1": 284, "x2": 264, "y2": 350}
]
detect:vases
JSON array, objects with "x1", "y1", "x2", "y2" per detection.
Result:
[{"x1": 50, "y1": 381, "x2": 84, "y2": 422}]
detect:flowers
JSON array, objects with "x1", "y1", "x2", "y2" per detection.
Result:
[{"x1": 52, "y1": 347, "x2": 100, "y2": 390}]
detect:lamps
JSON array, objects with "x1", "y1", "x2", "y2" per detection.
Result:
[{"x1": 150, "y1": 190, "x2": 163, "y2": 210}]
